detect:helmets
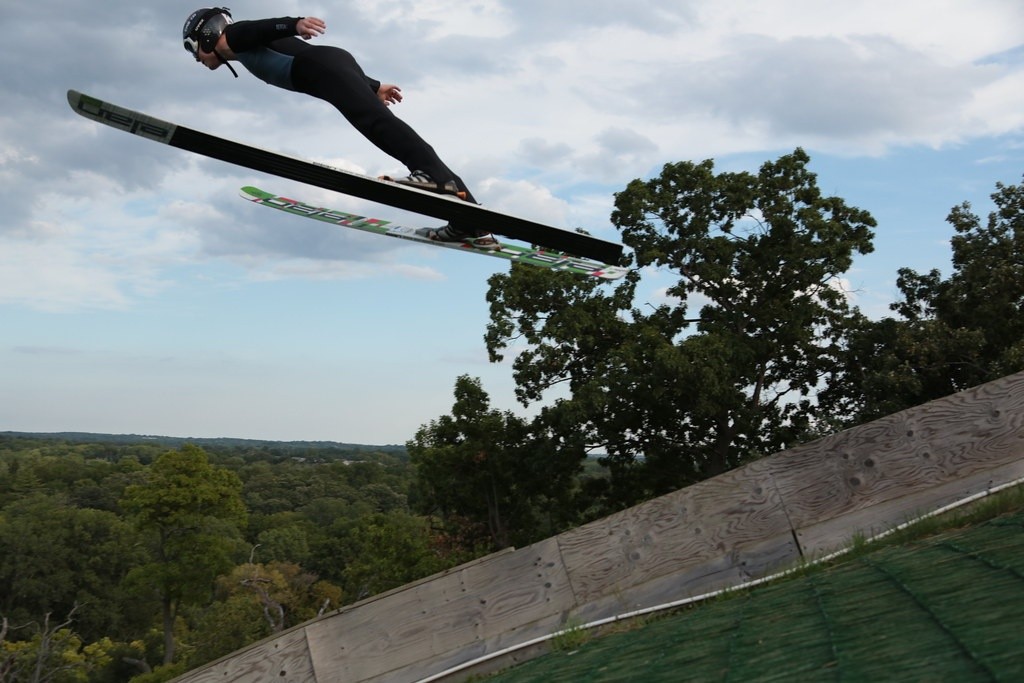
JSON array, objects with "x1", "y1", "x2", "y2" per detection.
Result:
[{"x1": 183, "y1": 7, "x2": 234, "y2": 54}]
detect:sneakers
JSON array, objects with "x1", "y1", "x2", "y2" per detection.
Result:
[
  {"x1": 378, "y1": 168, "x2": 458, "y2": 194},
  {"x1": 415, "y1": 220, "x2": 503, "y2": 252}
]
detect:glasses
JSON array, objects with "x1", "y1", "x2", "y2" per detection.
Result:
[{"x1": 183, "y1": 34, "x2": 199, "y2": 58}]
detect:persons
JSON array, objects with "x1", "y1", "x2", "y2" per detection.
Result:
[{"x1": 183, "y1": 6, "x2": 500, "y2": 252}]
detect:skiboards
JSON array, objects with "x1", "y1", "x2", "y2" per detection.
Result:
[{"x1": 64, "y1": 87, "x2": 632, "y2": 281}]
